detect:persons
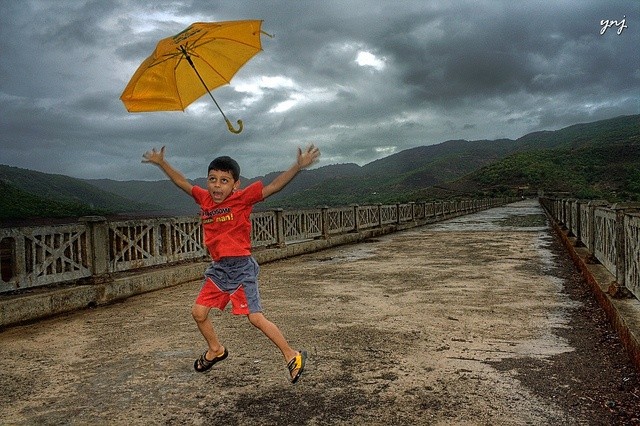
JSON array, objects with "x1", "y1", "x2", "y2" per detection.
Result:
[{"x1": 140, "y1": 143, "x2": 321, "y2": 384}]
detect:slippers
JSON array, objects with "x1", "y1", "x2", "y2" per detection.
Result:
[
  {"x1": 287, "y1": 350, "x2": 307, "y2": 384},
  {"x1": 195, "y1": 346, "x2": 228, "y2": 373}
]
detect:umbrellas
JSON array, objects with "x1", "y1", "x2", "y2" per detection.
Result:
[{"x1": 119, "y1": 18, "x2": 275, "y2": 134}]
